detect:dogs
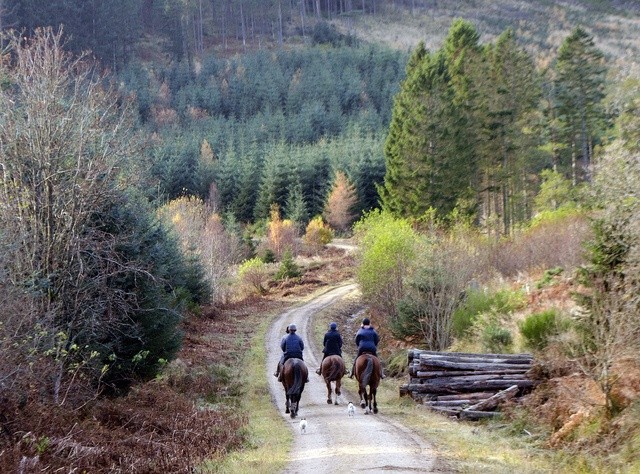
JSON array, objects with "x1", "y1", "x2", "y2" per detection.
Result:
[
  {"x1": 348, "y1": 401, "x2": 358, "y2": 417},
  {"x1": 300, "y1": 417, "x2": 308, "y2": 435}
]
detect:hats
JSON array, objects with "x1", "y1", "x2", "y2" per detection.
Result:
[{"x1": 363, "y1": 318, "x2": 370, "y2": 322}]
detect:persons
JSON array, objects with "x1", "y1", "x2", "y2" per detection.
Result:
[
  {"x1": 348, "y1": 319, "x2": 386, "y2": 379},
  {"x1": 274, "y1": 324, "x2": 309, "y2": 382},
  {"x1": 316, "y1": 322, "x2": 350, "y2": 376}
]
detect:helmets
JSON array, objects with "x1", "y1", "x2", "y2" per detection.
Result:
[
  {"x1": 288, "y1": 324, "x2": 297, "y2": 331},
  {"x1": 330, "y1": 322, "x2": 337, "y2": 327}
]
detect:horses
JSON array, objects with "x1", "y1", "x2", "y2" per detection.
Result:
[
  {"x1": 321, "y1": 354, "x2": 347, "y2": 405},
  {"x1": 281, "y1": 357, "x2": 310, "y2": 419},
  {"x1": 354, "y1": 352, "x2": 382, "y2": 414}
]
